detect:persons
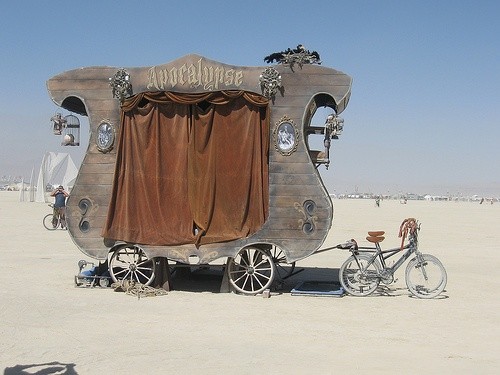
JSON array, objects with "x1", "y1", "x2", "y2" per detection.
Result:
[
  {"x1": 50, "y1": 185, "x2": 69, "y2": 228},
  {"x1": 377, "y1": 197, "x2": 380, "y2": 206}
]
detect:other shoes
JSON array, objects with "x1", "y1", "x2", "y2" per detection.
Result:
[
  {"x1": 61, "y1": 224, "x2": 64, "y2": 228},
  {"x1": 53, "y1": 225, "x2": 56, "y2": 228}
]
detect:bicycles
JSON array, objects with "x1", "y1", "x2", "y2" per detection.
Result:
[
  {"x1": 338, "y1": 218, "x2": 448, "y2": 298},
  {"x1": 43, "y1": 202, "x2": 68, "y2": 231}
]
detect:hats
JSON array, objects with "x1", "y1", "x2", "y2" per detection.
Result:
[{"x1": 59, "y1": 186, "x2": 64, "y2": 190}]
